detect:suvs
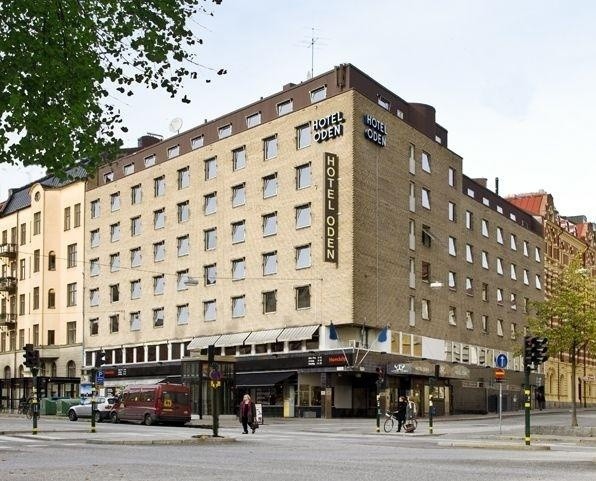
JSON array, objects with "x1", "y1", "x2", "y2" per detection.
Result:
[{"x1": 66, "y1": 396, "x2": 118, "y2": 423}]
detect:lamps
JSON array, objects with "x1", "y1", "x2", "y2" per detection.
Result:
[{"x1": 422, "y1": 274, "x2": 444, "y2": 288}]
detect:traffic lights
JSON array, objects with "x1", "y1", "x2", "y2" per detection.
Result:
[
  {"x1": 524, "y1": 336, "x2": 537, "y2": 367},
  {"x1": 22, "y1": 344, "x2": 32, "y2": 367},
  {"x1": 535, "y1": 338, "x2": 548, "y2": 366},
  {"x1": 96, "y1": 350, "x2": 105, "y2": 367},
  {"x1": 29, "y1": 351, "x2": 38, "y2": 369}
]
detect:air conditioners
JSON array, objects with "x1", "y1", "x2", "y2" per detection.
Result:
[{"x1": 349, "y1": 340, "x2": 361, "y2": 348}]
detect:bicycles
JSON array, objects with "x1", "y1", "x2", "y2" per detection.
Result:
[
  {"x1": 26, "y1": 403, "x2": 39, "y2": 421},
  {"x1": 383, "y1": 409, "x2": 417, "y2": 433}
]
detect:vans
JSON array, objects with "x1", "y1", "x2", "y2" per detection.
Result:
[{"x1": 108, "y1": 383, "x2": 192, "y2": 427}]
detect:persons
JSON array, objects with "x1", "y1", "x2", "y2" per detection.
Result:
[
  {"x1": 27, "y1": 391, "x2": 40, "y2": 413},
  {"x1": 393, "y1": 396, "x2": 406, "y2": 434},
  {"x1": 403, "y1": 395, "x2": 417, "y2": 433},
  {"x1": 236, "y1": 394, "x2": 260, "y2": 434}
]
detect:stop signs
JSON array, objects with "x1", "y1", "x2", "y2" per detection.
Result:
[{"x1": 495, "y1": 368, "x2": 505, "y2": 380}]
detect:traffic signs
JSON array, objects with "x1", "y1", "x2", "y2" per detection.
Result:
[{"x1": 95, "y1": 369, "x2": 103, "y2": 384}]
